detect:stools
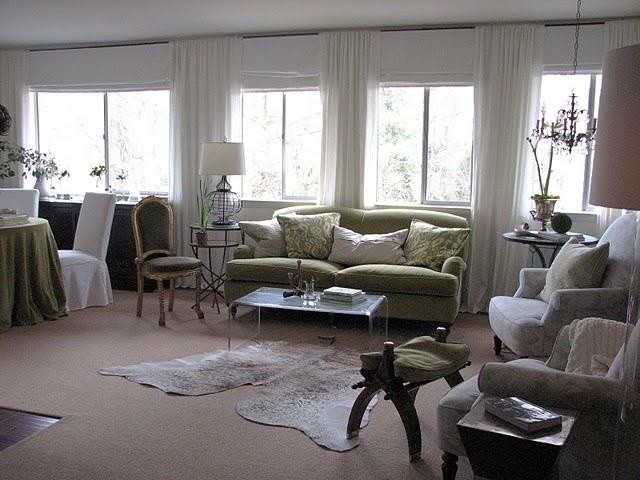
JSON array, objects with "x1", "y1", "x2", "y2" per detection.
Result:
[
  {"x1": 456, "y1": 395, "x2": 576, "y2": 480},
  {"x1": 345, "y1": 335, "x2": 472, "y2": 464}
]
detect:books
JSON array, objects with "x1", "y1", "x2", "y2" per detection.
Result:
[
  {"x1": 321, "y1": 286, "x2": 368, "y2": 305},
  {"x1": 537, "y1": 231, "x2": 585, "y2": 243},
  {"x1": 485, "y1": 396, "x2": 563, "y2": 431}
]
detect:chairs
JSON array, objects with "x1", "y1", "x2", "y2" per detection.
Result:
[
  {"x1": 0, "y1": 188, "x2": 40, "y2": 220},
  {"x1": 131, "y1": 194, "x2": 204, "y2": 327},
  {"x1": 57, "y1": 191, "x2": 117, "y2": 313}
]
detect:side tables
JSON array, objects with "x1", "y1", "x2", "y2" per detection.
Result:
[
  {"x1": 190, "y1": 220, "x2": 241, "y2": 314},
  {"x1": 502, "y1": 229, "x2": 599, "y2": 268}
]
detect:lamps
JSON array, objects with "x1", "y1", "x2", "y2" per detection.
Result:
[
  {"x1": 198, "y1": 136, "x2": 247, "y2": 227},
  {"x1": 589, "y1": 41, "x2": 639, "y2": 476}
]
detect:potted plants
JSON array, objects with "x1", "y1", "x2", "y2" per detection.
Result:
[
  {"x1": 525, "y1": 91, "x2": 597, "y2": 233},
  {"x1": 87, "y1": 160, "x2": 107, "y2": 190},
  {"x1": 0, "y1": 141, "x2": 70, "y2": 200}
]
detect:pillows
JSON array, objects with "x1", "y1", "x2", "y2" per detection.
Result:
[
  {"x1": 239, "y1": 218, "x2": 285, "y2": 258},
  {"x1": 277, "y1": 212, "x2": 341, "y2": 257},
  {"x1": 402, "y1": 217, "x2": 472, "y2": 272},
  {"x1": 536, "y1": 235, "x2": 610, "y2": 303},
  {"x1": 326, "y1": 224, "x2": 409, "y2": 266}
]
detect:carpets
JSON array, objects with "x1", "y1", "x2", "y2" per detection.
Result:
[{"x1": 97, "y1": 340, "x2": 380, "y2": 452}]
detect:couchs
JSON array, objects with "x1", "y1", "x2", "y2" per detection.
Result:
[
  {"x1": 437, "y1": 318, "x2": 640, "y2": 480},
  {"x1": 225, "y1": 206, "x2": 470, "y2": 336},
  {"x1": 489, "y1": 214, "x2": 638, "y2": 357}
]
